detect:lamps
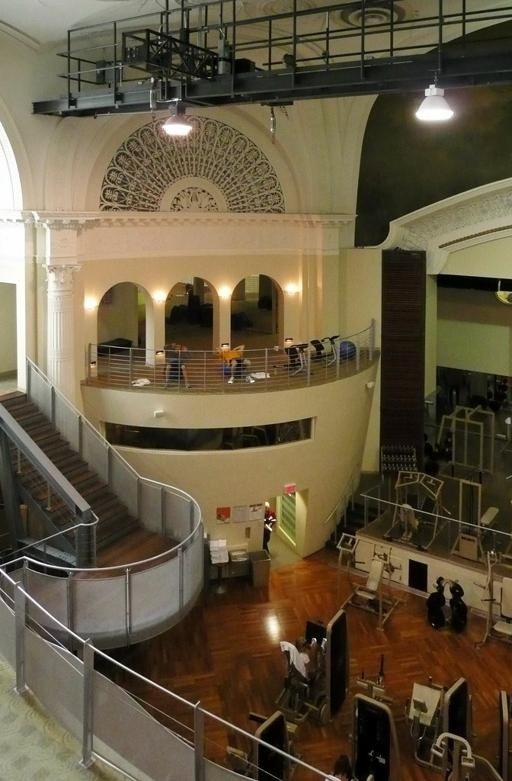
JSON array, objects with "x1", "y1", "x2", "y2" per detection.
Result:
[
  {"x1": 414, "y1": 84, "x2": 455, "y2": 124},
  {"x1": 162, "y1": 102, "x2": 193, "y2": 137}
]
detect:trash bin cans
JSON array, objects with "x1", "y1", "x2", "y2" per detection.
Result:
[{"x1": 249, "y1": 550, "x2": 272, "y2": 588}]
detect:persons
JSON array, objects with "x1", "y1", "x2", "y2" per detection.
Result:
[
  {"x1": 289, "y1": 636, "x2": 313, "y2": 682},
  {"x1": 422, "y1": 434, "x2": 434, "y2": 474},
  {"x1": 432, "y1": 577, "x2": 449, "y2": 606},
  {"x1": 164, "y1": 343, "x2": 192, "y2": 389},
  {"x1": 325, "y1": 754, "x2": 374, "y2": 781},
  {"x1": 487, "y1": 378, "x2": 510, "y2": 415},
  {"x1": 220, "y1": 343, "x2": 255, "y2": 384},
  {"x1": 264, "y1": 501, "x2": 277, "y2": 553}
]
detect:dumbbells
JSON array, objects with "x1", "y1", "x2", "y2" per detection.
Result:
[{"x1": 383, "y1": 444, "x2": 416, "y2": 471}]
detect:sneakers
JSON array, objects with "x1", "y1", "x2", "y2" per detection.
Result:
[
  {"x1": 228, "y1": 377, "x2": 234, "y2": 385},
  {"x1": 245, "y1": 376, "x2": 256, "y2": 384}
]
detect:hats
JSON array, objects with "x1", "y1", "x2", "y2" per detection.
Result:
[{"x1": 265, "y1": 501, "x2": 270, "y2": 508}]
